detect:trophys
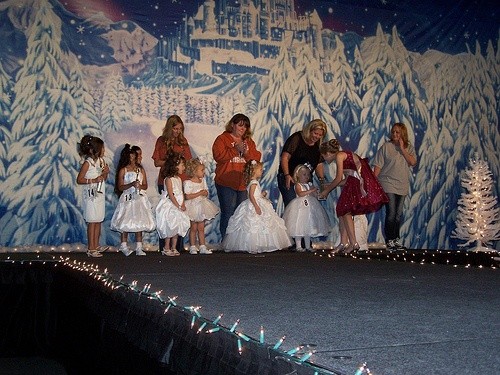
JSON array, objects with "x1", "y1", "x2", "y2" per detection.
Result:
[
  {"x1": 96, "y1": 163, "x2": 108, "y2": 193},
  {"x1": 134, "y1": 167, "x2": 143, "y2": 196},
  {"x1": 317, "y1": 175, "x2": 327, "y2": 201}
]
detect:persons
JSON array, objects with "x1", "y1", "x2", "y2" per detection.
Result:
[
  {"x1": 155, "y1": 151, "x2": 191, "y2": 257},
  {"x1": 293, "y1": 164, "x2": 327, "y2": 252},
  {"x1": 371, "y1": 122, "x2": 418, "y2": 250},
  {"x1": 151, "y1": 114, "x2": 193, "y2": 253},
  {"x1": 211, "y1": 113, "x2": 261, "y2": 253},
  {"x1": 319, "y1": 139, "x2": 390, "y2": 255},
  {"x1": 113, "y1": 142, "x2": 155, "y2": 255},
  {"x1": 183, "y1": 156, "x2": 221, "y2": 255},
  {"x1": 275, "y1": 119, "x2": 330, "y2": 250},
  {"x1": 241, "y1": 159, "x2": 268, "y2": 254},
  {"x1": 75, "y1": 134, "x2": 109, "y2": 258}
]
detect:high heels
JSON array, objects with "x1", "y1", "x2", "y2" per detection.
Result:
[{"x1": 331, "y1": 242, "x2": 360, "y2": 255}]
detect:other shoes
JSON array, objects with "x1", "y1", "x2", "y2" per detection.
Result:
[
  {"x1": 288, "y1": 243, "x2": 312, "y2": 251},
  {"x1": 224, "y1": 249, "x2": 230, "y2": 252},
  {"x1": 250, "y1": 251, "x2": 261, "y2": 255},
  {"x1": 179, "y1": 249, "x2": 189, "y2": 253}
]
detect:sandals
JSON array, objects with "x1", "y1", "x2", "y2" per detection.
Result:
[
  {"x1": 308, "y1": 248, "x2": 315, "y2": 252},
  {"x1": 95, "y1": 246, "x2": 109, "y2": 253},
  {"x1": 162, "y1": 248, "x2": 180, "y2": 256},
  {"x1": 86, "y1": 249, "x2": 103, "y2": 257},
  {"x1": 296, "y1": 248, "x2": 305, "y2": 252}
]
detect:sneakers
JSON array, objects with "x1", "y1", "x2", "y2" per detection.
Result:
[
  {"x1": 136, "y1": 248, "x2": 146, "y2": 255},
  {"x1": 200, "y1": 246, "x2": 212, "y2": 254},
  {"x1": 386, "y1": 237, "x2": 404, "y2": 251},
  {"x1": 122, "y1": 246, "x2": 133, "y2": 257},
  {"x1": 190, "y1": 246, "x2": 197, "y2": 254}
]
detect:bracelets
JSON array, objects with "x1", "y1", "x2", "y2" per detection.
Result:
[
  {"x1": 86, "y1": 178, "x2": 92, "y2": 184},
  {"x1": 284, "y1": 174, "x2": 290, "y2": 176},
  {"x1": 178, "y1": 205, "x2": 182, "y2": 209}
]
